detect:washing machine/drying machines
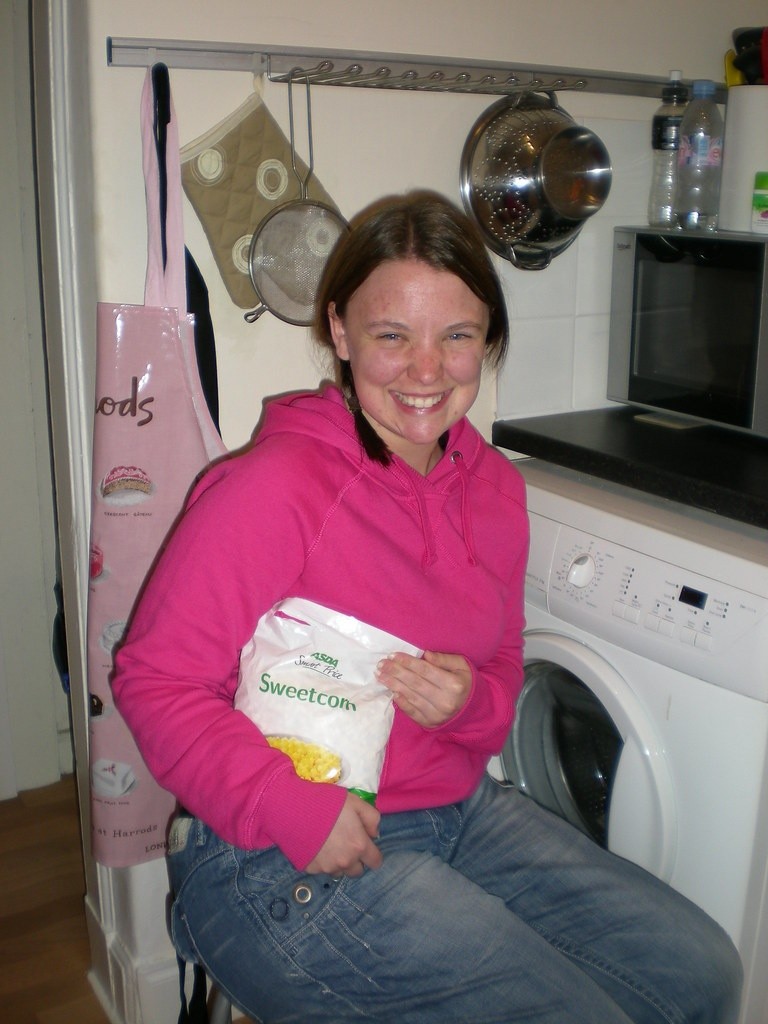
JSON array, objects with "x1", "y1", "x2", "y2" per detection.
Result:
[{"x1": 493, "y1": 456, "x2": 763, "y2": 1024}]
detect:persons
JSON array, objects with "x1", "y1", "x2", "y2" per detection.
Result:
[{"x1": 109, "y1": 188, "x2": 744, "y2": 1024}]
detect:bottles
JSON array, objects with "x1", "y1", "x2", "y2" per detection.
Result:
[
  {"x1": 676, "y1": 79, "x2": 724, "y2": 232},
  {"x1": 647, "y1": 86, "x2": 688, "y2": 228},
  {"x1": 751, "y1": 171, "x2": 768, "y2": 234}
]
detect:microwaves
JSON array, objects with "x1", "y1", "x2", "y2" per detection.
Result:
[{"x1": 606, "y1": 224, "x2": 768, "y2": 439}]
